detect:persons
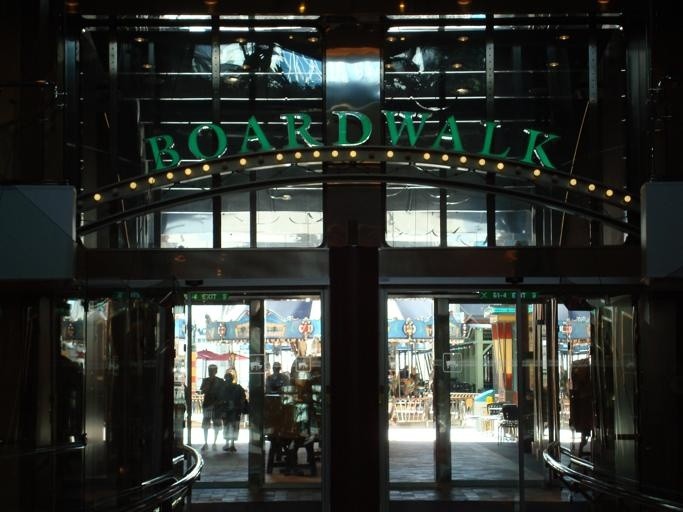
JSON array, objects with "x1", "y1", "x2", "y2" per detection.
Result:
[
  {"x1": 222, "y1": 372, "x2": 246, "y2": 451},
  {"x1": 266, "y1": 361, "x2": 289, "y2": 393},
  {"x1": 199, "y1": 364, "x2": 225, "y2": 450}
]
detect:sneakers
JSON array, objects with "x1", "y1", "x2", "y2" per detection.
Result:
[{"x1": 201, "y1": 444, "x2": 237, "y2": 453}]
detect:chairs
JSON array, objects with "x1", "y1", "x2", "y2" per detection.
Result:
[{"x1": 486, "y1": 400, "x2": 518, "y2": 446}]
